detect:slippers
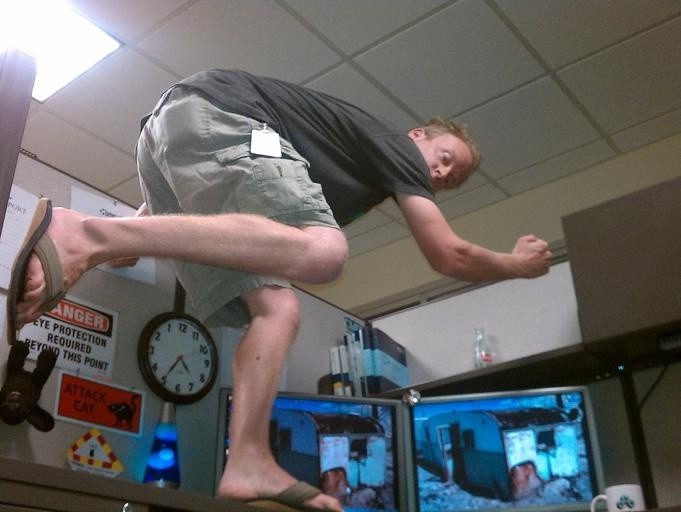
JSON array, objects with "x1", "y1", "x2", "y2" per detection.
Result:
[
  {"x1": 245, "y1": 481, "x2": 322, "y2": 512},
  {"x1": 7, "y1": 198, "x2": 65, "y2": 345}
]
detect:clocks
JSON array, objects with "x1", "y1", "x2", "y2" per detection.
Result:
[{"x1": 136, "y1": 308, "x2": 219, "y2": 405}]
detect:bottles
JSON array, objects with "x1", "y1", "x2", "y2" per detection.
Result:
[{"x1": 473, "y1": 327, "x2": 493, "y2": 369}]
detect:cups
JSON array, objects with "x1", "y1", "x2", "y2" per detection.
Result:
[{"x1": 591, "y1": 484, "x2": 647, "y2": 511}]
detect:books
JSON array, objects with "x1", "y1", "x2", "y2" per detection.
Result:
[{"x1": 329, "y1": 326, "x2": 408, "y2": 398}]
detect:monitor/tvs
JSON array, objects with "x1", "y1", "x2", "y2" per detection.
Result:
[
  {"x1": 213, "y1": 387, "x2": 407, "y2": 512},
  {"x1": 403, "y1": 385, "x2": 605, "y2": 512}
]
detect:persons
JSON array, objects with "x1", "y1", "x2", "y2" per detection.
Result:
[{"x1": 6, "y1": 68, "x2": 553, "y2": 512}]
